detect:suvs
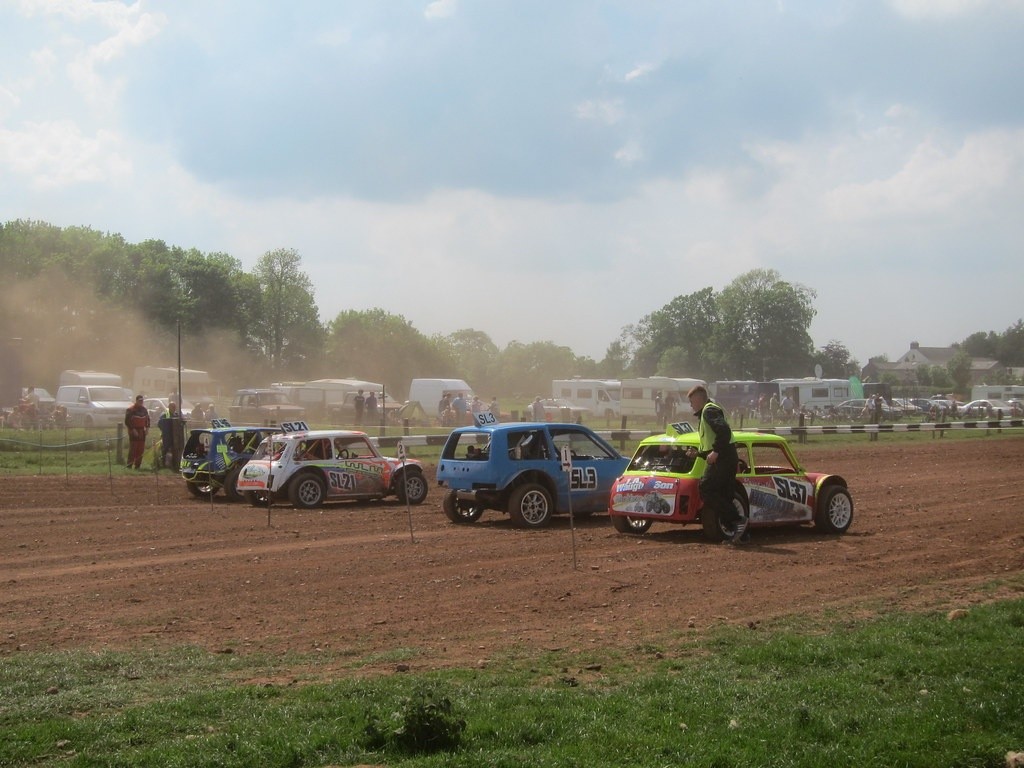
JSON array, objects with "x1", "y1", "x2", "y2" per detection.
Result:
[{"x1": 230, "y1": 389, "x2": 306, "y2": 422}]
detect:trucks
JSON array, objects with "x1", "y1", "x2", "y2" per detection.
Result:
[
  {"x1": 713, "y1": 380, "x2": 758, "y2": 411},
  {"x1": 132, "y1": 368, "x2": 215, "y2": 409},
  {"x1": 552, "y1": 379, "x2": 621, "y2": 418},
  {"x1": 620, "y1": 376, "x2": 716, "y2": 421},
  {"x1": 60, "y1": 370, "x2": 122, "y2": 386}
]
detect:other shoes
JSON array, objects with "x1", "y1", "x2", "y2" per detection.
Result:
[
  {"x1": 722, "y1": 535, "x2": 751, "y2": 545},
  {"x1": 732, "y1": 515, "x2": 749, "y2": 543}
]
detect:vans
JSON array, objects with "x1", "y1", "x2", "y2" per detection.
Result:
[
  {"x1": 54, "y1": 385, "x2": 138, "y2": 429},
  {"x1": 409, "y1": 378, "x2": 476, "y2": 416}
]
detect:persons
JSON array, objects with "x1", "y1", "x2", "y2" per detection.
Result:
[
  {"x1": 685, "y1": 386, "x2": 749, "y2": 548},
  {"x1": 531, "y1": 396, "x2": 547, "y2": 422},
  {"x1": 655, "y1": 390, "x2": 677, "y2": 427},
  {"x1": 0, "y1": 387, "x2": 74, "y2": 429},
  {"x1": 160, "y1": 388, "x2": 221, "y2": 467},
  {"x1": 354, "y1": 389, "x2": 500, "y2": 429},
  {"x1": 758, "y1": 393, "x2": 1023, "y2": 424},
  {"x1": 124, "y1": 394, "x2": 151, "y2": 470}
]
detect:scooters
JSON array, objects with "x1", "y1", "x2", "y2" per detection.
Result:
[{"x1": 800, "y1": 405, "x2": 845, "y2": 424}]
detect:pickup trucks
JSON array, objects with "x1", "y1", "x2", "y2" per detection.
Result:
[{"x1": 327, "y1": 392, "x2": 403, "y2": 424}]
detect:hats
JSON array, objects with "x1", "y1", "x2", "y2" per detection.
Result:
[{"x1": 136, "y1": 395, "x2": 144, "y2": 401}]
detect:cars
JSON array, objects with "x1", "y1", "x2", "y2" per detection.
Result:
[
  {"x1": 20, "y1": 387, "x2": 55, "y2": 409},
  {"x1": 931, "y1": 394, "x2": 1024, "y2": 417},
  {"x1": 435, "y1": 410, "x2": 631, "y2": 527},
  {"x1": 909, "y1": 399, "x2": 946, "y2": 415},
  {"x1": 236, "y1": 421, "x2": 429, "y2": 509},
  {"x1": 144, "y1": 397, "x2": 206, "y2": 425},
  {"x1": 440, "y1": 400, "x2": 510, "y2": 426},
  {"x1": 837, "y1": 400, "x2": 903, "y2": 422},
  {"x1": 879, "y1": 398, "x2": 922, "y2": 415},
  {"x1": 527, "y1": 398, "x2": 592, "y2": 421},
  {"x1": 180, "y1": 427, "x2": 306, "y2": 502},
  {"x1": 606, "y1": 421, "x2": 854, "y2": 544}
]
